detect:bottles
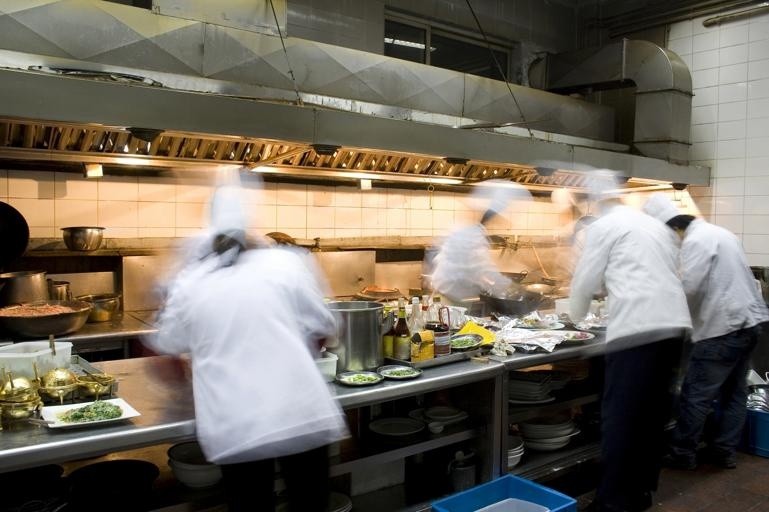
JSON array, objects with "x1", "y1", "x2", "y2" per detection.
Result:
[{"x1": 384, "y1": 295, "x2": 451, "y2": 361}]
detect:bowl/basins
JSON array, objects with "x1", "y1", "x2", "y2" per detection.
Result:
[
  {"x1": 167, "y1": 440, "x2": 222, "y2": 489},
  {"x1": 0, "y1": 226, "x2": 122, "y2": 339}
]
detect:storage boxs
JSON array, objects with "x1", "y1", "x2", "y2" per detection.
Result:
[
  {"x1": 745, "y1": 408, "x2": 769, "y2": 458},
  {"x1": 473, "y1": 498, "x2": 550, "y2": 512},
  {"x1": 431, "y1": 473, "x2": 576, "y2": 512}
]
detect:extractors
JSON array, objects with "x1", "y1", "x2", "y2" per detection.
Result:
[{"x1": 0, "y1": 1, "x2": 711, "y2": 195}]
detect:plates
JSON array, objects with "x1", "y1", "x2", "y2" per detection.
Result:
[
  {"x1": 507, "y1": 365, "x2": 599, "y2": 469},
  {"x1": 513, "y1": 317, "x2": 607, "y2": 345},
  {"x1": 329, "y1": 492, "x2": 355, "y2": 512},
  {"x1": 451, "y1": 334, "x2": 484, "y2": 352},
  {"x1": 334, "y1": 365, "x2": 423, "y2": 387},
  {"x1": 369, "y1": 405, "x2": 470, "y2": 436},
  {"x1": 39, "y1": 396, "x2": 141, "y2": 429}
]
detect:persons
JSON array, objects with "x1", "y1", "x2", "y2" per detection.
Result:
[{"x1": 140, "y1": 148, "x2": 769, "y2": 511}]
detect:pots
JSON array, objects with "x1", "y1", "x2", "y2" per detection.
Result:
[
  {"x1": 326, "y1": 300, "x2": 384, "y2": 372},
  {"x1": 481, "y1": 270, "x2": 562, "y2": 315}
]
